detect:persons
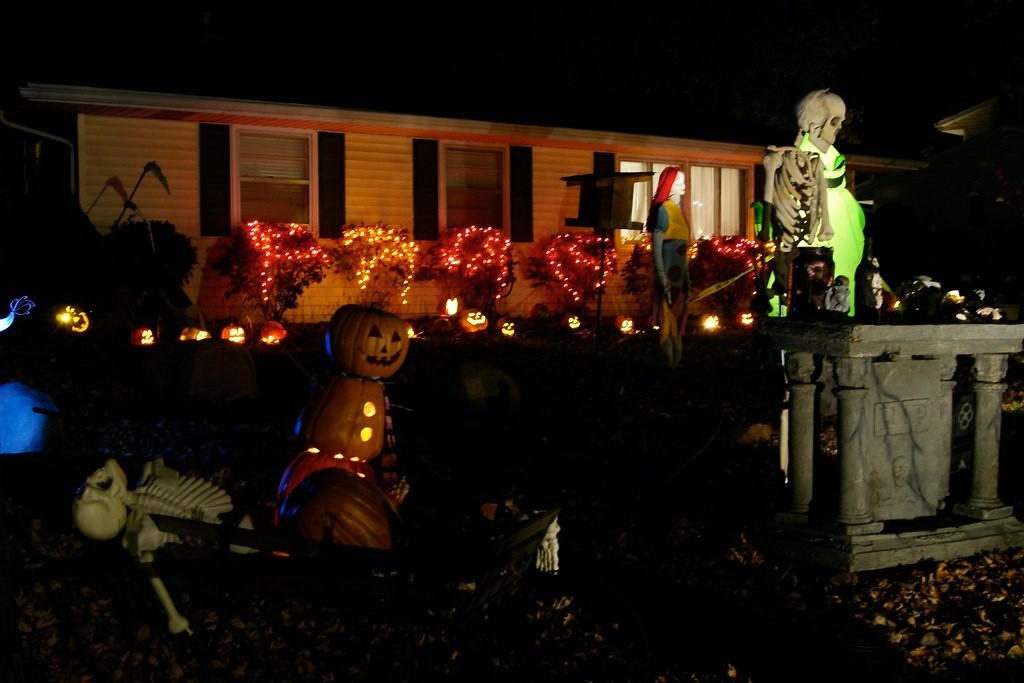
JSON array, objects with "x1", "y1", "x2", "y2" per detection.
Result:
[{"x1": 646, "y1": 168, "x2": 691, "y2": 367}]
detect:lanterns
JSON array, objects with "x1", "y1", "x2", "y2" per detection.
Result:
[{"x1": 329, "y1": 302, "x2": 409, "y2": 379}]
